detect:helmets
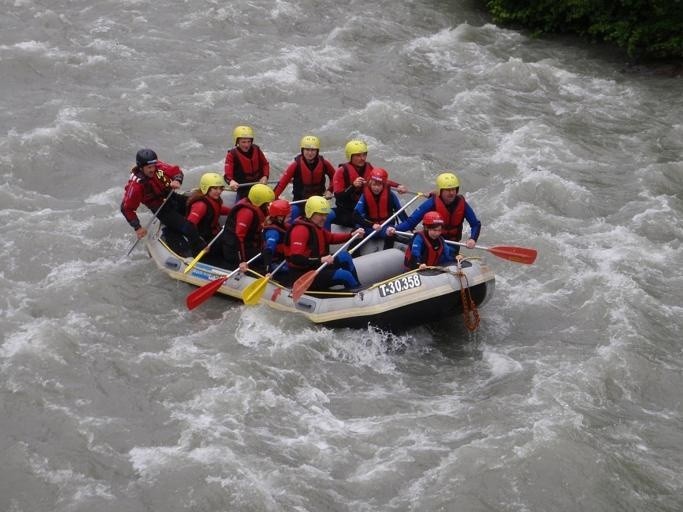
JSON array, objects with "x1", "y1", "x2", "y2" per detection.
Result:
[
  {"x1": 423, "y1": 212, "x2": 443, "y2": 224},
  {"x1": 200, "y1": 126, "x2": 388, "y2": 218},
  {"x1": 436, "y1": 173, "x2": 459, "y2": 197},
  {"x1": 136, "y1": 149, "x2": 158, "y2": 165}
]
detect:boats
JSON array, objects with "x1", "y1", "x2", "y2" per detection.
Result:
[{"x1": 146, "y1": 192, "x2": 495, "y2": 329}]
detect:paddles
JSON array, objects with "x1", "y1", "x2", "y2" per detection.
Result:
[
  {"x1": 293, "y1": 233, "x2": 360, "y2": 300},
  {"x1": 241, "y1": 260, "x2": 287, "y2": 304},
  {"x1": 187, "y1": 252, "x2": 262, "y2": 310},
  {"x1": 395, "y1": 231, "x2": 536, "y2": 264},
  {"x1": 185, "y1": 226, "x2": 225, "y2": 273}
]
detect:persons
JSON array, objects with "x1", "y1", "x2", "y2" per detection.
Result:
[
  {"x1": 120, "y1": 148, "x2": 189, "y2": 239},
  {"x1": 403, "y1": 211, "x2": 463, "y2": 274},
  {"x1": 348, "y1": 169, "x2": 408, "y2": 258},
  {"x1": 273, "y1": 136, "x2": 336, "y2": 227},
  {"x1": 326, "y1": 140, "x2": 406, "y2": 234},
  {"x1": 385, "y1": 173, "x2": 481, "y2": 262},
  {"x1": 179, "y1": 172, "x2": 362, "y2": 293},
  {"x1": 224, "y1": 126, "x2": 270, "y2": 204}
]
